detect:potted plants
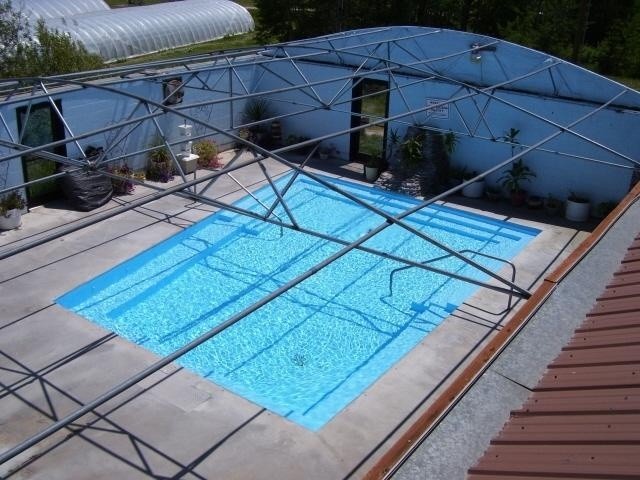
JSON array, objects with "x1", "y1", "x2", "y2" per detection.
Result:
[
  {"x1": 0, "y1": 190, "x2": 26, "y2": 231},
  {"x1": 110, "y1": 130, "x2": 175, "y2": 198},
  {"x1": 362, "y1": 129, "x2": 590, "y2": 221}
]
